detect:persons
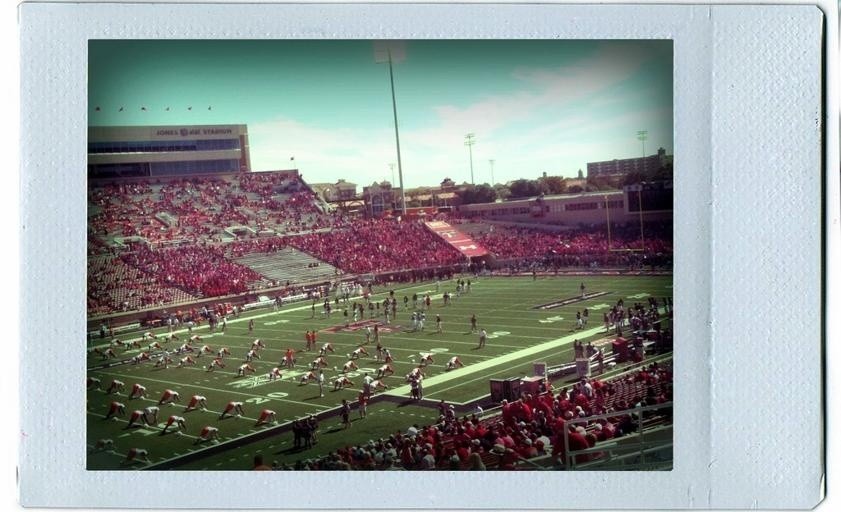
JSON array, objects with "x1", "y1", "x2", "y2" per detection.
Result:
[{"x1": 87, "y1": 172, "x2": 673, "y2": 470}]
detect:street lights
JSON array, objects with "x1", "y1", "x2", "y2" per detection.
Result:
[
  {"x1": 389, "y1": 163, "x2": 395, "y2": 188},
  {"x1": 463, "y1": 133, "x2": 475, "y2": 185},
  {"x1": 369, "y1": 40, "x2": 411, "y2": 217},
  {"x1": 489, "y1": 159, "x2": 494, "y2": 188},
  {"x1": 636, "y1": 129, "x2": 651, "y2": 194}
]
